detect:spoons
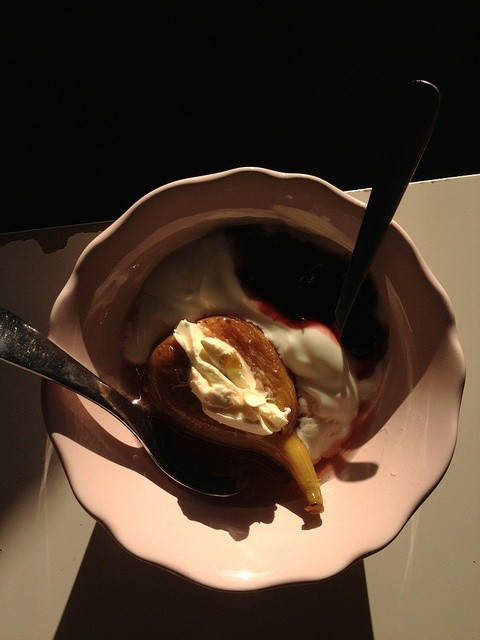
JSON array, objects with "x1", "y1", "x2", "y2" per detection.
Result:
[{"x1": 0, "y1": 303, "x2": 251, "y2": 498}]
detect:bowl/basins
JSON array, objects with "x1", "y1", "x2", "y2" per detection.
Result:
[{"x1": 39, "y1": 166, "x2": 467, "y2": 595}]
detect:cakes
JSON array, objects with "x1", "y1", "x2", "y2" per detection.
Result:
[{"x1": 116, "y1": 230, "x2": 390, "y2": 517}]
